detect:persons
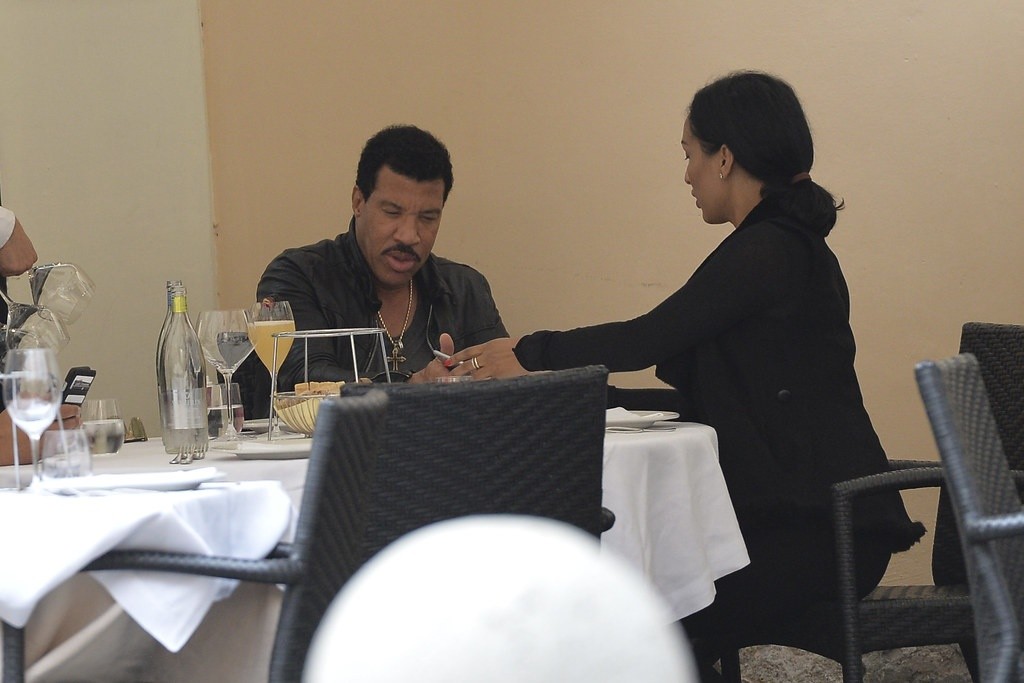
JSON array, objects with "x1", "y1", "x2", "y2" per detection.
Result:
[
  {"x1": 232, "y1": 122, "x2": 512, "y2": 422},
  {"x1": 443, "y1": 67, "x2": 926, "y2": 683},
  {"x1": 0, "y1": 188, "x2": 83, "y2": 468}
]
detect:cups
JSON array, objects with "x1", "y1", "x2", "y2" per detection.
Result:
[
  {"x1": 206, "y1": 383, "x2": 245, "y2": 441},
  {"x1": 436, "y1": 375, "x2": 472, "y2": 383},
  {"x1": 42, "y1": 430, "x2": 93, "y2": 494},
  {"x1": 79, "y1": 398, "x2": 125, "y2": 456}
]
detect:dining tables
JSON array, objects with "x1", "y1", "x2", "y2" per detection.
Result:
[{"x1": 2, "y1": 420, "x2": 751, "y2": 683}]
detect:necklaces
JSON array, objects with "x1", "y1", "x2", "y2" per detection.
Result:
[{"x1": 377, "y1": 278, "x2": 413, "y2": 370}]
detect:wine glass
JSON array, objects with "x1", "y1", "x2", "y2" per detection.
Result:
[
  {"x1": 1, "y1": 349, "x2": 63, "y2": 499},
  {"x1": 247, "y1": 300, "x2": 306, "y2": 440},
  {"x1": 0, "y1": 290, "x2": 70, "y2": 376},
  {"x1": 194, "y1": 309, "x2": 259, "y2": 451},
  {"x1": 27, "y1": 261, "x2": 97, "y2": 324}
]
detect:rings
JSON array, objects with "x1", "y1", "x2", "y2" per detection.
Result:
[{"x1": 472, "y1": 357, "x2": 480, "y2": 370}]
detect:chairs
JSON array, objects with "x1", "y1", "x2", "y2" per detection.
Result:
[
  {"x1": 915, "y1": 354, "x2": 1024, "y2": 683},
  {"x1": 4, "y1": 364, "x2": 618, "y2": 682},
  {"x1": 718, "y1": 323, "x2": 1024, "y2": 683}
]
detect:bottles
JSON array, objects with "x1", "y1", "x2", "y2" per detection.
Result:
[{"x1": 156, "y1": 280, "x2": 209, "y2": 453}]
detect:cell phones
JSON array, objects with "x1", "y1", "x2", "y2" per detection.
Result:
[
  {"x1": 433, "y1": 349, "x2": 464, "y2": 364},
  {"x1": 60, "y1": 365, "x2": 97, "y2": 405}
]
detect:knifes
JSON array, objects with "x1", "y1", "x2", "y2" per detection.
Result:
[{"x1": 607, "y1": 427, "x2": 677, "y2": 433}]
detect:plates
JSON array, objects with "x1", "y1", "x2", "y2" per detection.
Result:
[
  {"x1": 38, "y1": 464, "x2": 227, "y2": 495},
  {"x1": 606, "y1": 410, "x2": 681, "y2": 427},
  {"x1": 243, "y1": 417, "x2": 297, "y2": 431},
  {"x1": 229, "y1": 444, "x2": 311, "y2": 460}
]
